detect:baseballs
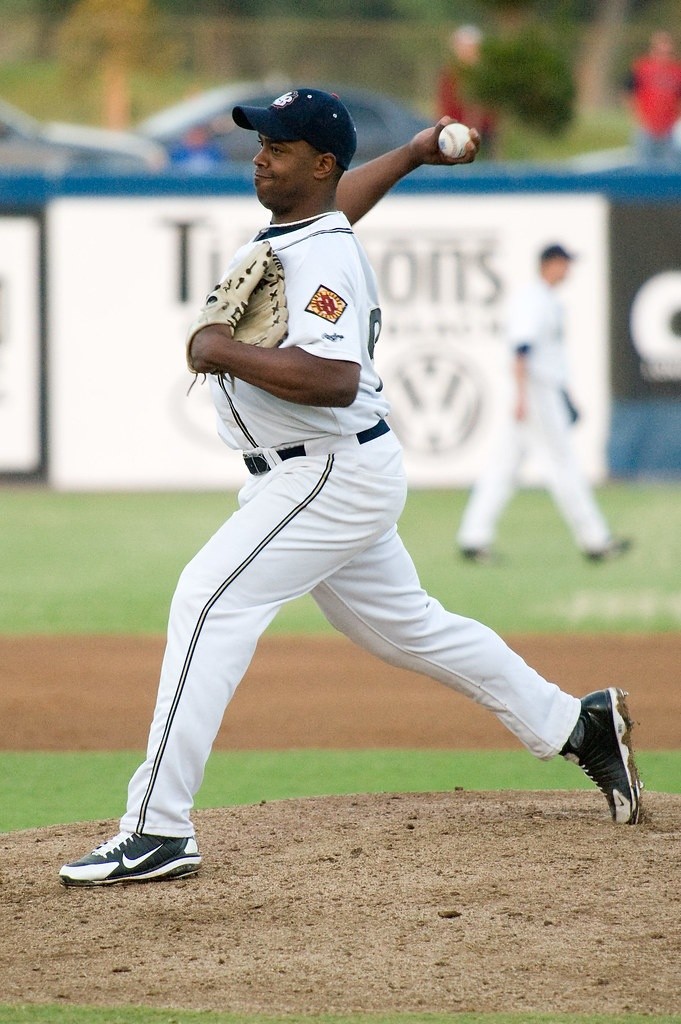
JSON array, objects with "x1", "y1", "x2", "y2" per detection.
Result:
[{"x1": 437, "y1": 122, "x2": 472, "y2": 159}]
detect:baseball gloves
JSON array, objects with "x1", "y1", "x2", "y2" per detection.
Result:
[{"x1": 189, "y1": 240, "x2": 291, "y2": 362}]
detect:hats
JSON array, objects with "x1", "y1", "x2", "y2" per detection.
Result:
[
  {"x1": 542, "y1": 244, "x2": 574, "y2": 263},
  {"x1": 231, "y1": 88, "x2": 358, "y2": 171}
]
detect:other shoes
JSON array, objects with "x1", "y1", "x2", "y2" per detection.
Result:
[
  {"x1": 586, "y1": 537, "x2": 628, "y2": 560},
  {"x1": 458, "y1": 547, "x2": 490, "y2": 565}
]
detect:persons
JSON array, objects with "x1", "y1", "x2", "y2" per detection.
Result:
[
  {"x1": 460, "y1": 245, "x2": 633, "y2": 563},
  {"x1": 59, "y1": 89, "x2": 640, "y2": 887}
]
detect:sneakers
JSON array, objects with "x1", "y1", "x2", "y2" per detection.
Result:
[
  {"x1": 558, "y1": 686, "x2": 646, "y2": 825},
  {"x1": 57, "y1": 831, "x2": 204, "y2": 887}
]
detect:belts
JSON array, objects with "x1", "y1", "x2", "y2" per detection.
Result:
[{"x1": 242, "y1": 418, "x2": 390, "y2": 476}]
